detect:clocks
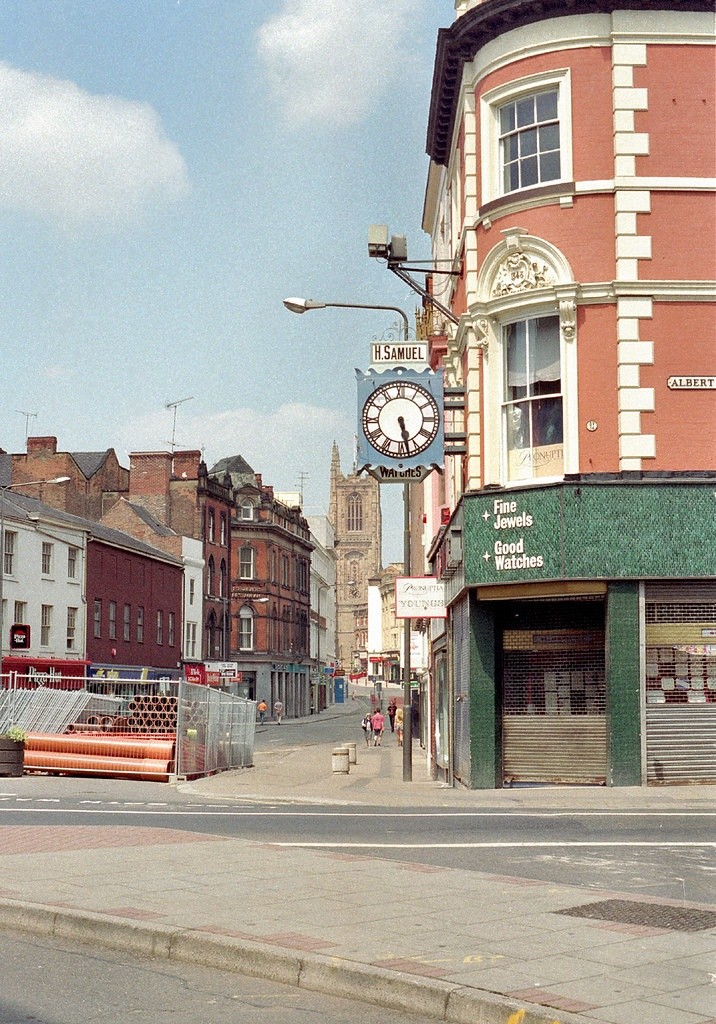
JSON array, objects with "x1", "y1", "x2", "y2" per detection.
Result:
[{"x1": 353, "y1": 367, "x2": 449, "y2": 477}]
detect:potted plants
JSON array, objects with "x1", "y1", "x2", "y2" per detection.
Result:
[{"x1": 0, "y1": 724, "x2": 29, "y2": 777}]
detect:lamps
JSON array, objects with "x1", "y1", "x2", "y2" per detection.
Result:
[
  {"x1": 367, "y1": 223, "x2": 463, "y2": 276},
  {"x1": 387, "y1": 236, "x2": 460, "y2": 326}
]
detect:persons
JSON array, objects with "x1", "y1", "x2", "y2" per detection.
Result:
[
  {"x1": 394, "y1": 708, "x2": 403, "y2": 746},
  {"x1": 258, "y1": 700, "x2": 267, "y2": 725},
  {"x1": 274, "y1": 699, "x2": 283, "y2": 725},
  {"x1": 386, "y1": 699, "x2": 397, "y2": 732},
  {"x1": 362, "y1": 708, "x2": 384, "y2": 746}
]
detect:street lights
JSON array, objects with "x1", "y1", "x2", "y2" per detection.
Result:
[
  {"x1": 0, "y1": 476, "x2": 72, "y2": 688},
  {"x1": 282, "y1": 297, "x2": 413, "y2": 782},
  {"x1": 316, "y1": 581, "x2": 354, "y2": 714},
  {"x1": 220, "y1": 596, "x2": 270, "y2": 692}
]
